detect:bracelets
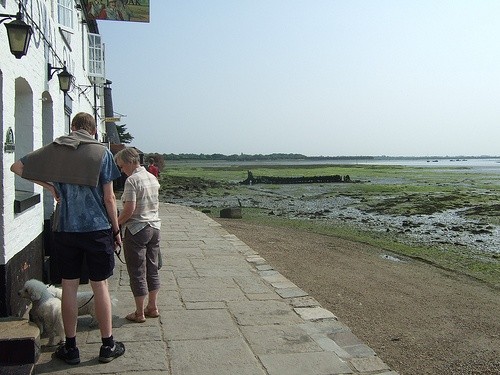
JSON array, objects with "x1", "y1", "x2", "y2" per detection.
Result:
[{"x1": 113, "y1": 230, "x2": 120, "y2": 238}]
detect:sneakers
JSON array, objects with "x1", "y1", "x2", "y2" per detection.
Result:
[
  {"x1": 100, "y1": 340, "x2": 125, "y2": 363},
  {"x1": 55, "y1": 343, "x2": 80, "y2": 365}
]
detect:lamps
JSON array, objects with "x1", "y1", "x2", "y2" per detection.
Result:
[
  {"x1": 48, "y1": 61, "x2": 73, "y2": 96},
  {"x1": 0, "y1": 3, "x2": 34, "y2": 59}
]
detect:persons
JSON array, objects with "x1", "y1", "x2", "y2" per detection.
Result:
[
  {"x1": 9, "y1": 112, "x2": 126, "y2": 364},
  {"x1": 146, "y1": 158, "x2": 159, "y2": 179},
  {"x1": 116, "y1": 148, "x2": 161, "y2": 323}
]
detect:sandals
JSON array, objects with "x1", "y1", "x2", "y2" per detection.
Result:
[
  {"x1": 125, "y1": 311, "x2": 147, "y2": 323},
  {"x1": 143, "y1": 307, "x2": 160, "y2": 317}
]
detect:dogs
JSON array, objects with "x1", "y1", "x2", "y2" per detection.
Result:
[
  {"x1": 46, "y1": 283, "x2": 119, "y2": 328},
  {"x1": 18, "y1": 279, "x2": 66, "y2": 348}
]
